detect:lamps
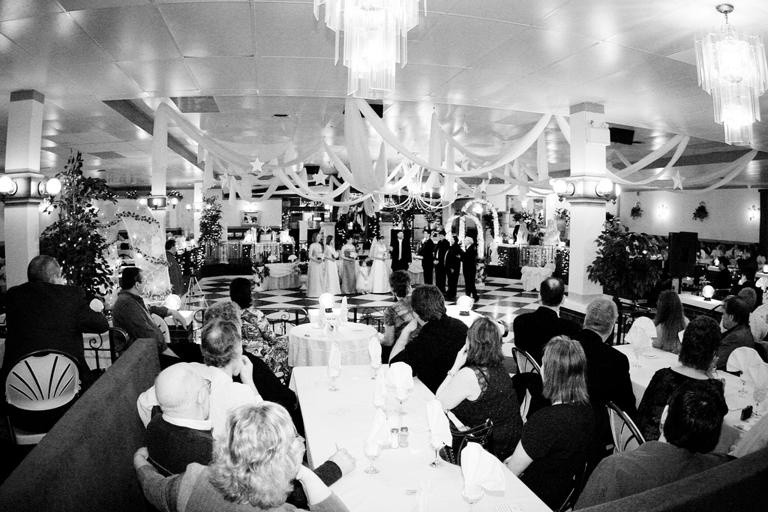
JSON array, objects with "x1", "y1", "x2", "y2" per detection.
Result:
[
  {"x1": 690, "y1": 3, "x2": 768, "y2": 150},
  {"x1": 551, "y1": 172, "x2": 578, "y2": 202},
  {"x1": 0, "y1": 168, "x2": 64, "y2": 206},
  {"x1": 594, "y1": 176, "x2": 624, "y2": 203},
  {"x1": 312, "y1": 0, "x2": 428, "y2": 103},
  {"x1": 134, "y1": 193, "x2": 181, "y2": 215}
]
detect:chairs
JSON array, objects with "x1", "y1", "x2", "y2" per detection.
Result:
[
  {"x1": 605, "y1": 394, "x2": 646, "y2": 455},
  {"x1": 513, "y1": 345, "x2": 548, "y2": 404},
  {"x1": 507, "y1": 367, "x2": 535, "y2": 426},
  {"x1": 5, "y1": 348, "x2": 85, "y2": 446}
]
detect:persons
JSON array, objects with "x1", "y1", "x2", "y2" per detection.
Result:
[
  {"x1": 513, "y1": 221, "x2": 768, "y2": 510},
  {"x1": 437, "y1": 318, "x2": 524, "y2": 458},
  {"x1": 0, "y1": 222, "x2": 354, "y2": 512},
  {"x1": 305, "y1": 216, "x2": 541, "y2": 391}
]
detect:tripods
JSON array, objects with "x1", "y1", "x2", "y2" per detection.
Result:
[{"x1": 184, "y1": 274, "x2": 209, "y2": 310}]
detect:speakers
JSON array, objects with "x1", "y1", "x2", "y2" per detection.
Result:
[
  {"x1": 669, "y1": 232, "x2": 697, "y2": 277},
  {"x1": 610, "y1": 127, "x2": 635, "y2": 145},
  {"x1": 303, "y1": 163, "x2": 320, "y2": 174},
  {"x1": 343, "y1": 104, "x2": 383, "y2": 118}
]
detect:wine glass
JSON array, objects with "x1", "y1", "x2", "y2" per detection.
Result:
[{"x1": 323, "y1": 352, "x2": 487, "y2": 512}]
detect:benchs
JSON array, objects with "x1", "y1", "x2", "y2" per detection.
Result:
[
  {"x1": 3, "y1": 338, "x2": 162, "y2": 510},
  {"x1": 550, "y1": 437, "x2": 768, "y2": 511}
]
